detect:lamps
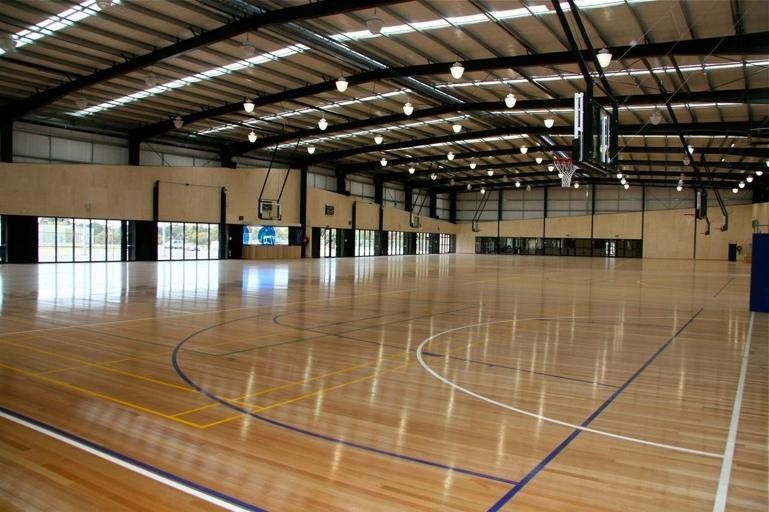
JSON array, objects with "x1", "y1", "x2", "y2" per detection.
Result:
[
  {"x1": 244, "y1": 94, "x2": 255, "y2": 114},
  {"x1": 402, "y1": 97, "x2": 414, "y2": 116},
  {"x1": 173, "y1": 115, "x2": 183, "y2": 128},
  {"x1": 452, "y1": 121, "x2": 462, "y2": 134},
  {"x1": 75, "y1": 89, "x2": 88, "y2": 109},
  {"x1": 731, "y1": 168, "x2": 764, "y2": 195},
  {"x1": 535, "y1": 152, "x2": 543, "y2": 164},
  {"x1": 2, "y1": 24, "x2": 18, "y2": 53},
  {"x1": 450, "y1": 62, "x2": 465, "y2": 80},
  {"x1": 650, "y1": 105, "x2": 663, "y2": 124},
  {"x1": 239, "y1": 32, "x2": 255, "y2": 58},
  {"x1": 505, "y1": 86, "x2": 516, "y2": 109},
  {"x1": 409, "y1": 151, "x2": 530, "y2": 194},
  {"x1": 618, "y1": 171, "x2": 629, "y2": 190},
  {"x1": 543, "y1": 111, "x2": 554, "y2": 129},
  {"x1": 248, "y1": 129, "x2": 257, "y2": 143},
  {"x1": 94, "y1": 1, "x2": 115, "y2": 9},
  {"x1": 676, "y1": 142, "x2": 694, "y2": 192},
  {"x1": 574, "y1": 181, "x2": 580, "y2": 189},
  {"x1": 144, "y1": 67, "x2": 157, "y2": 87},
  {"x1": 374, "y1": 133, "x2": 384, "y2": 145},
  {"x1": 595, "y1": 48, "x2": 613, "y2": 67},
  {"x1": 336, "y1": 70, "x2": 348, "y2": 92},
  {"x1": 365, "y1": 7, "x2": 384, "y2": 36},
  {"x1": 380, "y1": 158, "x2": 388, "y2": 167},
  {"x1": 307, "y1": 141, "x2": 315, "y2": 154},
  {"x1": 318, "y1": 113, "x2": 328, "y2": 130},
  {"x1": 520, "y1": 145, "x2": 528, "y2": 155},
  {"x1": 548, "y1": 164, "x2": 554, "y2": 172}
]
